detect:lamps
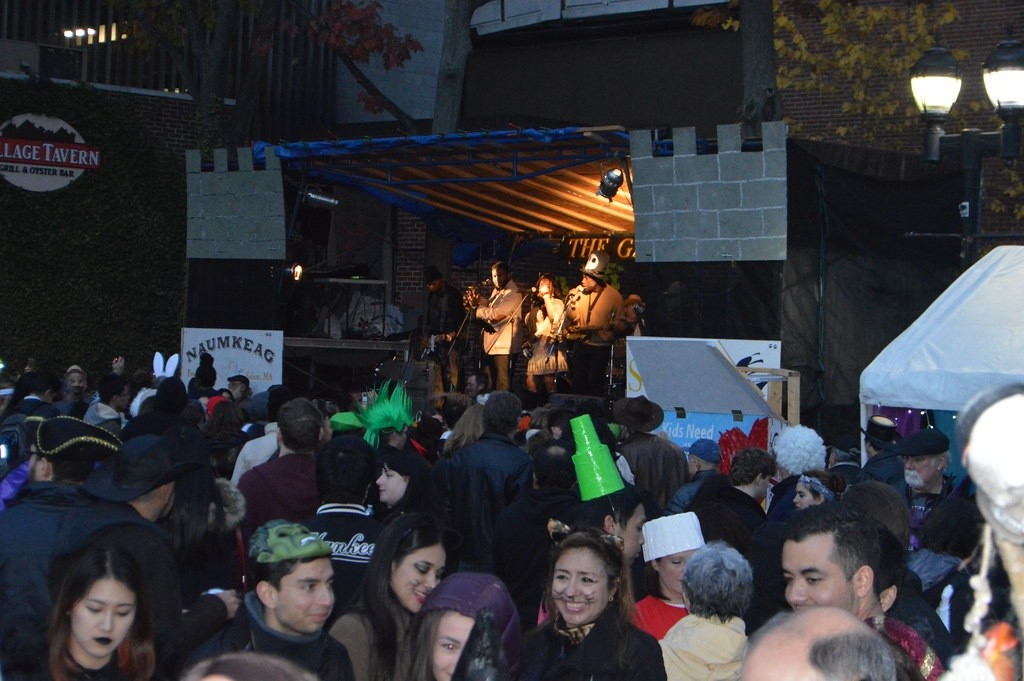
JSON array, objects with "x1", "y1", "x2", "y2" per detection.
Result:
[
  {"x1": 596, "y1": 158, "x2": 625, "y2": 201},
  {"x1": 269, "y1": 261, "x2": 304, "y2": 280},
  {"x1": 307, "y1": 188, "x2": 341, "y2": 211}
]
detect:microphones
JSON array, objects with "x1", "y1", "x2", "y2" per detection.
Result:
[
  {"x1": 518, "y1": 287, "x2": 536, "y2": 293},
  {"x1": 476, "y1": 279, "x2": 492, "y2": 288},
  {"x1": 570, "y1": 285, "x2": 584, "y2": 297}
]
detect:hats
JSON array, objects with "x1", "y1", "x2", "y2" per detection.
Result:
[
  {"x1": 614, "y1": 394, "x2": 665, "y2": 433},
  {"x1": 893, "y1": 428, "x2": 950, "y2": 456},
  {"x1": 578, "y1": 249, "x2": 610, "y2": 281},
  {"x1": 64, "y1": 364, "x2": 85, "y2": 376},
  {"x1": 227, "y1": 375, "x2": 250, "y2": 389},
  {"x1": 641, "y1": 512, "x2": 707, "y2": 561},
  {"x1": 856, "y1": 415, "x2": 899, "y2": 447},
  {"x1": 682, "y1": 438, "x2": 722, "y2": 463},
  {"x1": 422, "y1": 265, "x2": 441, "y2": 283},
  {"x1": 354, "y1": 377, "x2": 414, "y2": 447},
  {"x1": 960, "y1": 393, "x2": 1024, "y2": 548},
  {"x1": 120, "y1": 460, "x2": 178, "y2": 499},
  {"x1": 157, "y1": 376, "x2": 187, "y2": 405},
  {"x1": 98, "y1": 372, "x2": 127, "y2": 403},
  {"x1": 23, "y1": 413, "x2": 124, "y2": 465}
]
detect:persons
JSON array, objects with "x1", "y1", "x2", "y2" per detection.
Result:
[
  {"x1": 608, "y1": 295, "x2": 648, "y2": 390},
  {"x1": 524, "y1": 276, "x2": 569, "y2": 393},
  {"x1": 0, "y1": 352, "x2": 1022, "y2": 681},
  {"x1": 466, "y1": 261, "x2": 525, "y2": 391},
  {"x1": 562, "y1": 250, "x2": 625, "y2": 394},
  {"x1": 421, "y1": 266, "x2": 468, "y2": 393}
]
